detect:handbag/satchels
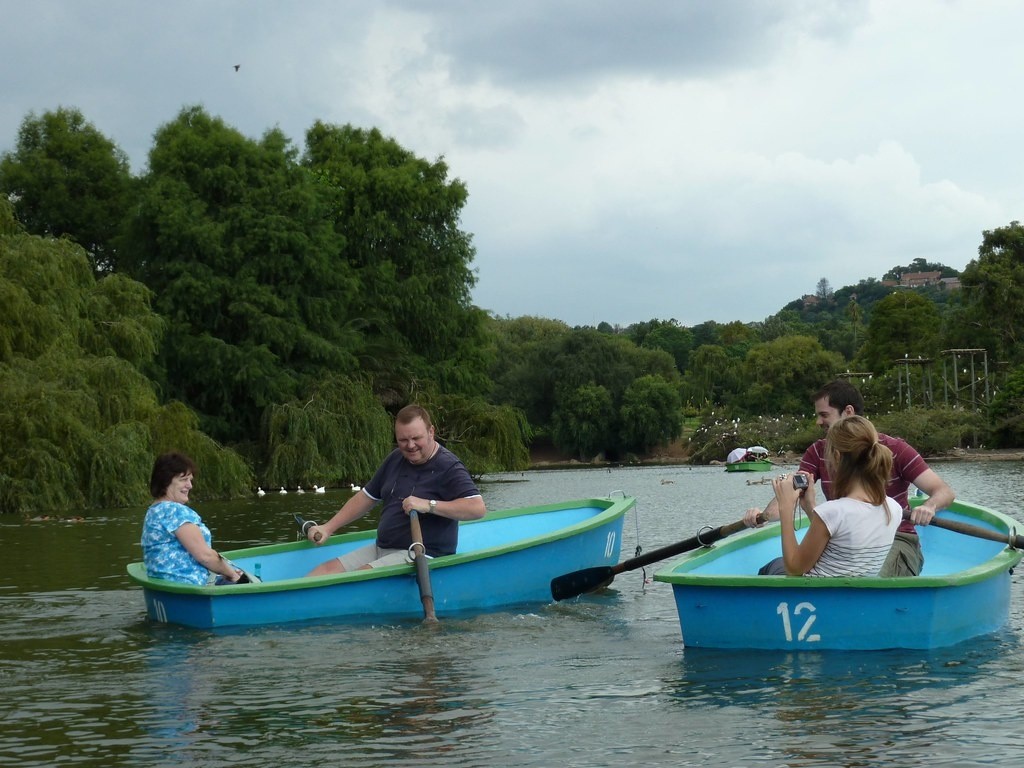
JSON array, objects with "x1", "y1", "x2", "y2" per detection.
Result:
[{"x1": 215, "y1": 573, "x2": 262, "y2": 586}]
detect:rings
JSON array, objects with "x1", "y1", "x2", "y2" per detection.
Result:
[{"x1": 779, "y1": 474, "x2": 787, "y2": 481}]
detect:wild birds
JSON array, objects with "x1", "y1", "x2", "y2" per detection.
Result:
[
  {"x1": 659, "y1": 478, "x2": 675, "y2": 486},
  {"x1": 256, "y1": 484, "x2": 362, "y2": 496},
  {"x1": 744, "y1": 475, "x2": 773, "y2": 486}
]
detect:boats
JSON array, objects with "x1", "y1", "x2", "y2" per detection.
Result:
[
  {"x1": 654, "y1": 488, "x2": 1024, "y2": 653},
  {"x1": 724, "y1": 458, "x2": 772, "y2": 472},
  {"x1": 125, "y1": 488, "x2": 639, "y2": 629}
]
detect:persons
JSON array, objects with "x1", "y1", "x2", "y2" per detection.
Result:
[
  {"x1": 758, "y1": 416, "x2": 902, "y2": 578},
  {"x1": 742, "y1": 380, "x2": 955, "y2": 579},
  {"x1": 737, "y1": 452, "x2": 770, "y2": 462},
  {"x1": 306, "y1": 405, "x2": 486, "y2": 579},
  {"x1": 141, "y1": 452, "x2": 243, "y2": 586}
]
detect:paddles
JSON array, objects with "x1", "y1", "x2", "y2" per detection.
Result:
[
  {"x1": 902, "y1": 509, "x2": 1024, "y2": 552},
  {"x1": 550, "y1": 511, "x2": 770, "y2": 602},
  {"x1": 410, "y1": 510, "x2": 439, "y2": 625},
  {"x1": 294, "y1": 514, "x2": 323, "y2": 542},
  {"x1": 763, "y1": 459, "x2": 779, "y2": 466}
]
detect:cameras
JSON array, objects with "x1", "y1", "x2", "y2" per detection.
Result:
[{"x1": 791, "y1": 474, "x2": 809, "y2": 491}]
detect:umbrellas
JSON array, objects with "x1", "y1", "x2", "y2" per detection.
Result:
[
  {"x1": 748, "y1": 446, "x2": 769, "y2": 456},
  {"x1": 727, "y1": 448, "x2": 746, "y2": 463}
]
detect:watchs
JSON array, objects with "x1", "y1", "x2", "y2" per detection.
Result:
[{"x1": 428, "y1": 500, "x2": 436, "y2": 513}]
